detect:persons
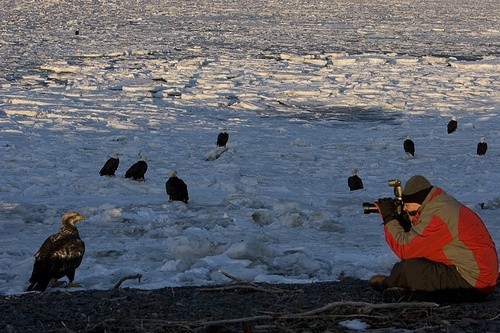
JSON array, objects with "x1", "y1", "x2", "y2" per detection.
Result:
[{"x1": 368, "y1": 175, "x2": 499, "y2": 304}]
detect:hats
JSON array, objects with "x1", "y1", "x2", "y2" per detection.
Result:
[{"x1": 403, "y1": 175, "x2": 433, "y2": 197}]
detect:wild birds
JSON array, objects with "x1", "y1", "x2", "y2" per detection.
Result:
[
  {"x1": 99, "y1": 152, "x2": 123, "y2": 177},
  {"x1": 215, "y1": 131, "x2": 229, "y2": 147},
  {"x1": 477, "y1": 137, "x2": 488, "y2": 156},
  {"x1": 165, "y1": 170, "x2": 190, "y2": 204},
  {"x1": 124, "y1": 154, "x2": 148, "y2": 183},
  {"x1": 348, "y1": 169, "x2": 364, "y2": 191},
  {"x1": 403, "y1": 135, "x2": 415, "y2": 158},
  {"x1": 447, "y1": 116, "x2": 457, "y2": 134}
]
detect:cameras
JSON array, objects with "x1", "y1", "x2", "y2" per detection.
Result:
[{"x1": 363, "y1": 180, "x2": 403, "y2": 219}]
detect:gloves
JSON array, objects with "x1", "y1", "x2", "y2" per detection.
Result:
[{"x1": 374, "y1": 196, "x2": 399, "y2": 226}]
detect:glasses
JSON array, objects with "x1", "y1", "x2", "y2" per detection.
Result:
[{"x1": 406, "y1": 205, "x2": 422, "y2": 216}]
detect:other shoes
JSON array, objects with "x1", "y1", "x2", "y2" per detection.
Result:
[{"x1": 370, "y1": 275, "x2": 407, "y2": 303}]
